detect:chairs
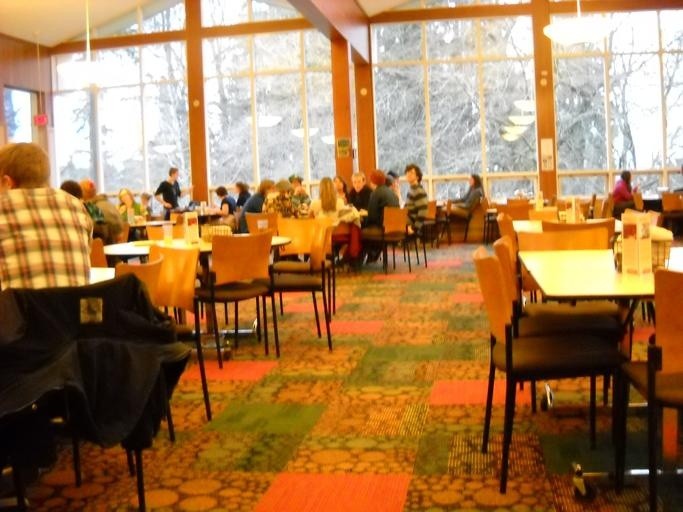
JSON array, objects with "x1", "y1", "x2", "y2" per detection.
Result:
[
  {"x1": 491, "y1": 235, "x2": 623, "y2": 415},
  {"x1": 332, "y1": 220, "x2": 358, "y2": 276},
  {"x1": 528, "y1": 208, "x2": 558, "y2": 221},
  {"x1": 89, "y1": 238, "x2": 108, "y2": 268},
  {"x1": 567, "y1": 201, "x2": 591, "y2": 221},
  {"x1": 360, "y1": 205, "x2": 411, "y2": 274},
  {"x1": 277, "y1": 217, "x2": 336, "y2": 322},
  {"x1": 147, "y1": 244, "x2": 212, "y2": 442},
  {"x1": 593, "y1": 199, "x2": 604, "y2": 219},
  {"x1": 616, "y1": 266, "x2": 683, "y2": 512},
  {"x1": 663, "y1": 191, "x2": 683, "y2": 240},
  {"x1": 93, "y1": 220, "x2": 130, "y2": 245},
  {"x1": 497, "y1": 212, "x2": 517, "y2": 251},
  {"x1": 589, "y1": 194, "x2": 596, "y2": 218},
  {"x1": 6, "y1": 273, "x2": 147, "y2": 512},
  {"x1": 245, "y1": 212, "x2": 278, "y2": 263},
  {"x1": 633, "y1": 192, "x2": 644, "y2": 211},
  {"x1": 471, "y1": 244, "x2": 631, "y2": 496},
  {"x1": 480, "y1": 200, "x2": 497, "y2": 245},
  {"x1": 441, "y1": 195, "x2": 482, "y2": 242},
  {"x1": 602, "y1": 199, "x2": 614, "y2": 218},
  {"x1": 393, "y1": 225, "x2": 427, "y2": 270},
  {"x1": 424, "y1": 200, "x2": 439, "y2": 249},
  {"x1": 194, "y1": 236, "x2": 280, "y2": 369},
  {"x1": 648, "y1": 210, "x2": 665, "y2": 228},
  {"x1": 507, "y1": 198, "x2": 529, "y2": 209},
  {"x1": 146, "y1": 225, "x2": 184, "y2": 241},
  {"x1": 115, "y1": 252, "x2": 175, "y2": 452},
  {"x1": 551, "y1": 194, "x2": 557, "y2": 207},
  {"x1": 263, "y1": 228, "x2": 333, "y2": 355}
]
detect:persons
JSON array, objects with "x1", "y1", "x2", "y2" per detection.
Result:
[
  {"x1": 404, "y1": 164, "x2": 428, "y2": 237},
  {"x1": 207, "y1": 186, "x2": 237, "y2": 225},
  {"x1": 60, "y1": 180, "x2": 105, "y2": 225},
  {"x1": 441, "y1": 174, "x2": 484, "y2": 222},
  {"x1": 236, "y1": 181, "x2": 251, "y2": 207},
  {"x1": 0, "y1": 143, "x2": 94, "y2": 291},
  {"x1": 155, "y1": 168, "x2": 194, "y2": 220},
  {"x1": 289, "y1": 174, "x2": 311, "y2": 210},
  {"x1": 117, "y1": 187, "x2": 141, "y2": 224},
  {"x1": 79, "y1": 178, "x2": 124, "y2": 245},
  {"x1": 333, "y1": 176, "x2": 347, "y2": 206},
  {"x1": 141, "y1": 192, "x2": 154, "y2": 216},
  {"x1": 309, "y1": 177, "x2": 345, "y2": 223},
  {"x1": 239, "y1": 180, "x2": 276, "y2": 234},
  {"x1": 362, "y1": 170, "x2": 399, "y2": 237},
  {"x1": 347, "y1": 172, "x2": 372, "y2": 212},
  {"x1": 612, "y1": 170, "x2": 639, "y2": 214},
  {"x1": 263, "y1": 179, "x2": 301, "y2": 220}
]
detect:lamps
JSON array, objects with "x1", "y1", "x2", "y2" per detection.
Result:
[{"x1": 34, "y1": 32, "x2": 48, "y2": 126}]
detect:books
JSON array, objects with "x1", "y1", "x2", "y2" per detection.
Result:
[
  {"x1": 184, "y1": 211, "x2": 199, "y2": 241},
  {"x1": 621, "y1": 213, "x2": 652, "y2": 276},
  {"x1": 565, "y1": 197, "x2": 580, "y2": 224}
]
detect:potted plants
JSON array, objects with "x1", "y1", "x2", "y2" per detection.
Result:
[
  {"x1": 273, "y1": 180, "x2": 295, "y2": 218},
  {"x1": 288, "y1": 175, "x2": 304, "y2": 185},
  {"x1": 289, "y1": 194, "x2": 310, "y2": 218}
]
